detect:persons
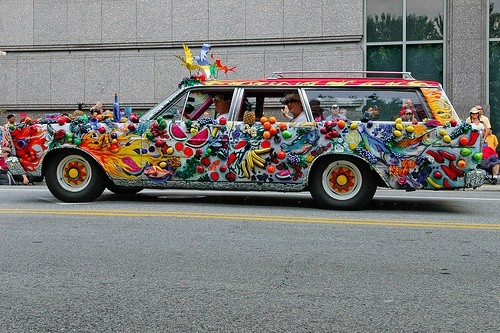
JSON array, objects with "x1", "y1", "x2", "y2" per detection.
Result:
[
  {"x1": 309, "y1": 100, "x2": 324, "y2": 120},
  {"x1": 3, "y1": 114, "x2": 18, "y2": 154},
  {"x1": 466, "y1": 105, "x2": 500, "y2": 174},
  {"x1": 360, "y1": 105, "x2": 379, "y2": 121},
  {"x1": 402, "y1": 110, "x2": 412, "y2": 121},
  {"x1": 214, "y1": 92, "x2": 233, "y2": 121},
  {"x1": 326, "y1": 105, "x2": 347, "y2": 121},
  {"x1": 281, "y1": 93, "x2": 307, "y2": 122},
  {"x1": 0, "y1": 147, "x2": 12, "y2": 174}
]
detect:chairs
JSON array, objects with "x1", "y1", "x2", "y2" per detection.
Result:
[
  {"x1": 310, "y1": 100, "x2": 322, "y2": 120},
  {"x1": 228, "y1": 95, "x2": 252, "y2": 121}
]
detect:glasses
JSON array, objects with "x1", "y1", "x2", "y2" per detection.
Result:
[
  {"x1": 213, "y1": 97, "x2": 224, "y2": 102},
  {"x1": 285, "y1": 99, "x2": 298, "y2": 105},
  {"x1": 406, "y1": 113, "x2": 412, "y2": 115}
]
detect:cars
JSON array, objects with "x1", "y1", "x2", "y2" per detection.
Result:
[{"x1": 0, "y1": 69, "x2": 486, "y2": 210}]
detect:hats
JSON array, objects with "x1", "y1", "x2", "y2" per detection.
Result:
[
  {"x1": 280, "y1": 93, "x2": 300, "y2": 104},
  {"x1": 332, "y1": 105, "x2": 338, "y2": 108},
  {"x1": 469, "y1": 108, "x2": 479, "y2": 114}
]
